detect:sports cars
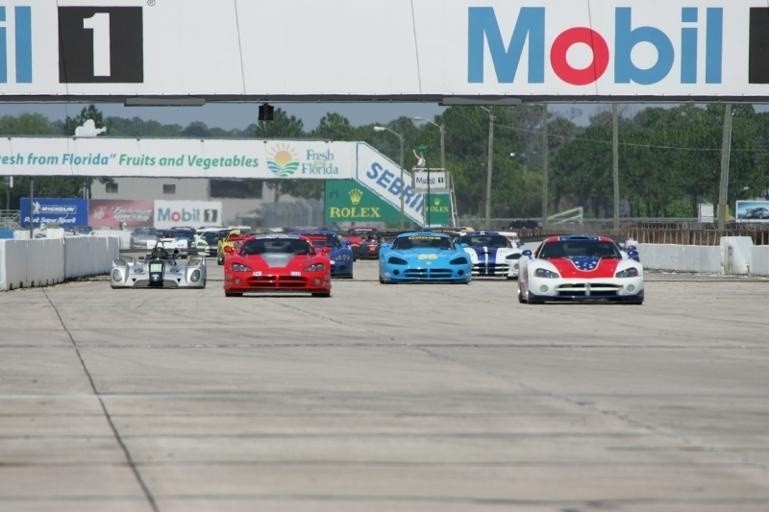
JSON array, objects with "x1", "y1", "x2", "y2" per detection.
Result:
[{"x1": 110, "y1": 242, "x2": 207, "y2": 288}]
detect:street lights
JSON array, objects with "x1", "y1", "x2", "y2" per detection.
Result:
[
  {"x1": 415, "y1": 114, "x2": 444, "y2": 169},
  {"x1": 375, "y1": 125, "x2": 403, "y2": 228}
]
